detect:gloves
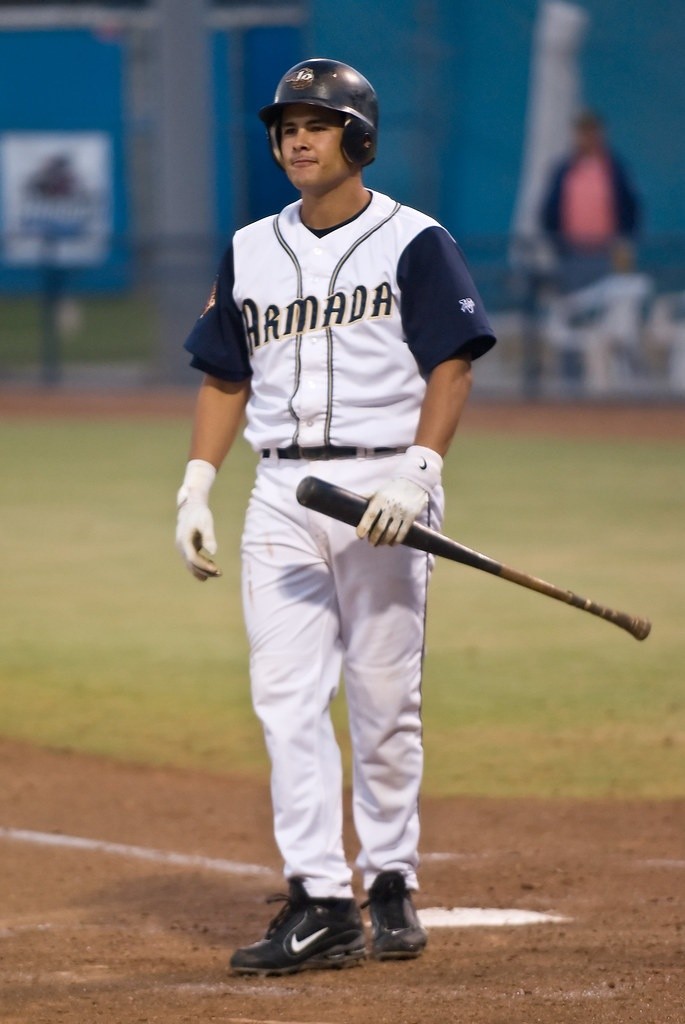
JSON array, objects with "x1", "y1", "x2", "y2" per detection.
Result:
[
  {"x1": 175, "y1": 498, "x2": 222, "y2": 581},
  {"x1": 357, "y1": 476, "x2": 428, "y2": 549}
]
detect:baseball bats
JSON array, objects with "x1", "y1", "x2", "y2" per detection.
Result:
[{"x1": 294, "y1": 474, "x2": 654, "y2": 642}]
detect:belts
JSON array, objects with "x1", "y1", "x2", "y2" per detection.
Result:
[{"x1": 261, "y1": 446, "x2": 396, "y2": 460}]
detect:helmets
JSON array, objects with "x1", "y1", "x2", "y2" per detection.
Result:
[{"x1": 259, "y1": 58, "x2": 379, "y2": 167}]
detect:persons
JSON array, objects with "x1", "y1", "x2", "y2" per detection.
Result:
[
  {"x1": 174, "y1": 57, "x2": 497, "y2": 973},
  {"x1": 534, "y1": 104, "x2": 638, "y2": 383}
]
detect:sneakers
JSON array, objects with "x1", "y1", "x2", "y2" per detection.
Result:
[
  {"x1": 361, "y1": 871, "x2": 427, "y2": 959},
  {"x1": 230, "y1": 879, "x2": 366, "y2": 974}
]
